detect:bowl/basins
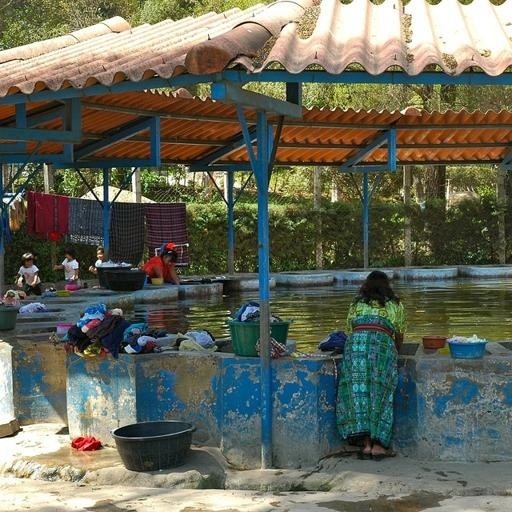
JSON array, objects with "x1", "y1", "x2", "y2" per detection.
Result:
[
  {"x1": 152, "y1": 278, "x2": 164, "y2": 285},
  {"x1": 0, "y1": 306, "x2": 19, "y2": 330},
  {"x1": 423, "y1": 335, "x2": 447, "y2": 349},
  {"x1": 95, "y1": 266, "x2": 132, "y2": 286},
  {"x1": 447, "y1": 340, "x2": 486, "y2": 358},
  {"x1": 227, "y1": 320, "x2": 292, "y2": 356},
  {"x1": 396, "y1": 341, "x2": 419, "y2": 355},
  {"x1": 111, "y1": 420, "x2": 196, "y2": 471},
  {"x1": 104, "y1": 270, "x2": 148, "y2": 290}
]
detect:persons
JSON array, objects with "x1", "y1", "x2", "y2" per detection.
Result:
[
  {"x1": 90, "y1": 247, "x2": 105, "y2": 274},
  {"x1": 15, "y1": 253, "x2": 44, "y2": 296},
  {"x1": 143, "y1": 241, "x2": 180, "y2": 285},
  {"x1": 54, "y1": 248, "x2": 80, "y2": 285},
  {"x1": 335, "y1": 270, "x2": 406, "y2": 459}
]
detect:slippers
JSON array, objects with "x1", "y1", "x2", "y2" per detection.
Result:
[{"x1": 361, "y1": 449, "x2": 396, "y2": 461}]
